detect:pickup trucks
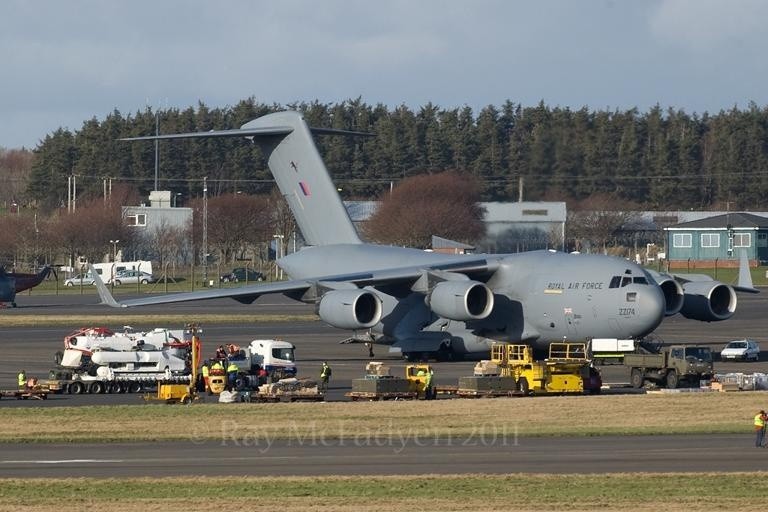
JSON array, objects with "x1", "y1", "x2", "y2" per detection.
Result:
[{"x1": 656, "y1": 251, "x2": 666, "y2": 261}]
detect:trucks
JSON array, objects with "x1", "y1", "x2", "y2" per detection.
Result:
[
  {"x1": 624, "y1": 345, "x2": 716, "y2": 388},
  {"x1": 404, "y1": 365, "x2": 439, "y2": 401}
]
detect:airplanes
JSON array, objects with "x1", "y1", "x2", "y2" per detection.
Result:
[{"x1": 85, "y1": 108, "x2": 760, "y2": 364}]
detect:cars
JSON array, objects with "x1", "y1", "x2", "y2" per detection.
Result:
[
  {"x1": 719, "y1": 336, "x2": 760, "y2": 363},
  {"x1": 113, "y1": 270, "x2": 155, "y2": 286},
  {"x1": 219, "y1": 268, "x2": 267, "y2": 282},
  {"x1": 63, "y1": 273, "x2": 96, "y2": 287},
  {"x1": 646, "y1": 254, "x2": 654, "y2": 264},
  {"x1": 109, "y1": 270, "x2": 136, "y2": 285}
]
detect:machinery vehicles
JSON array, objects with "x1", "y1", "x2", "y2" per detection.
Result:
[
  {"x1": 52, "y1": 321, "x2": 296, "y2": 388},
  {"x1": 458, "y1": 343, "x2": 602, "y2": 396},
  {"x1": 204, "y1": 367, "x2": 229, "y2": 395}
]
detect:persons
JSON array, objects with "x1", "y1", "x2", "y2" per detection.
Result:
[
  {"x1": 229, "y1": 371, "x2": 237, "y2": 387},
  {"x1": 216, "y1": 346, "x2": 226, "y2": 357},
  {"x1": 754, "y1": 410, "x2": 768, "y2": 447},
  {"x1": 19, "y1": 369, "x2": 36, "y2": 388},
  {"x1": 422, "y1": 373, "x2": 432, "y2": 400},
  {"x1": 320, "y1": 361, "x2": 329, "y2": 383},
  {"x1": 417, "y1": 369, "x2": 425, "y2": 376}
]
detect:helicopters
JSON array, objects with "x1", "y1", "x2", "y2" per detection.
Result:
[{"x1": 0, "y1": 262, "x2": 64, "y2": 309}]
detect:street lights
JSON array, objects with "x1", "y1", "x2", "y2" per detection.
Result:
[
  {"x1": 11, "y1": 203, "x2": 19, "y2": 213},
  {"x1": 110, "y1": 239, "x2": 120, "y2": 285},
  {"x1": 203, "y1": 175, "x2": 212, "y2": 275},
  {"x1": 173, "y1": 191, "x2": 183, "y2": 206},
  {"x1": 271, "y1": 233, "x2": 285, "y2": 279}
]
